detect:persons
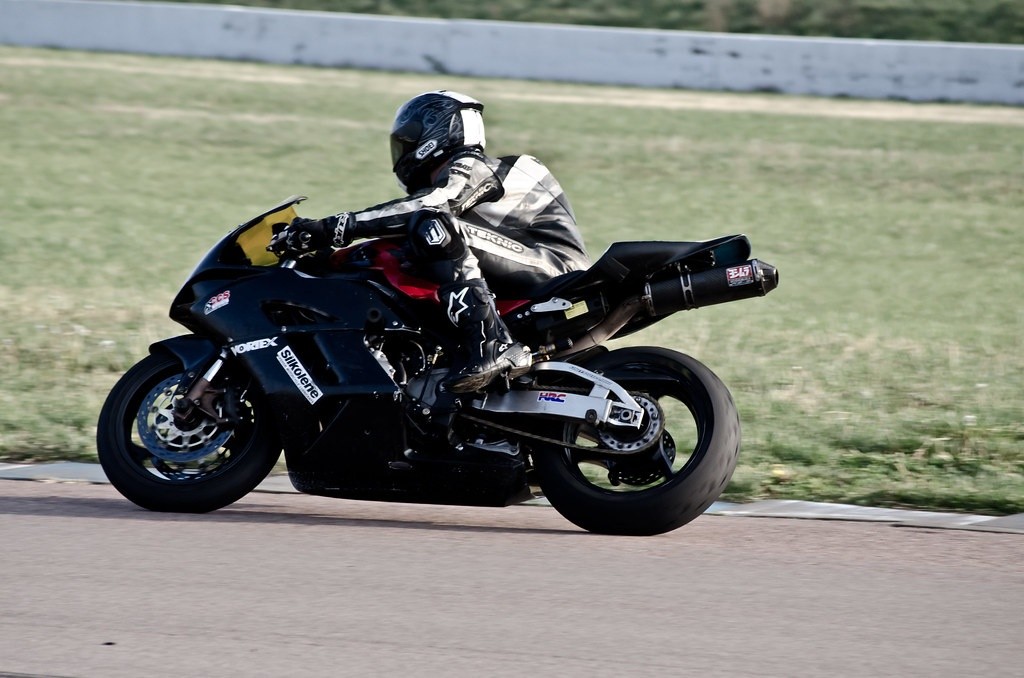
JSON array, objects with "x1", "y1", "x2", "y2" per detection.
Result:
[{"x1": 268, "y1": 89, "x2": 593, "y2": 396}]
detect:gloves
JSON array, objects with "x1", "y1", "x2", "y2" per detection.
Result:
[{"x1": 265, "y1": 211, "x2": 356, "y2": 262}]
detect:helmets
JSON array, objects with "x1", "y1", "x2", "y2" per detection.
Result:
[{"x1": 390, "y1": 90, "x2": 486, "y2": 196}]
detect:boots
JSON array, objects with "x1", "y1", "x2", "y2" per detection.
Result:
[{"x1": 437, "y1": 278, "x2": 533, "y2": 394}]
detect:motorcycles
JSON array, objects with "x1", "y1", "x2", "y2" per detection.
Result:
[{"x1": 94, "y1": 193, "x2": 778, "y2": 535}]
